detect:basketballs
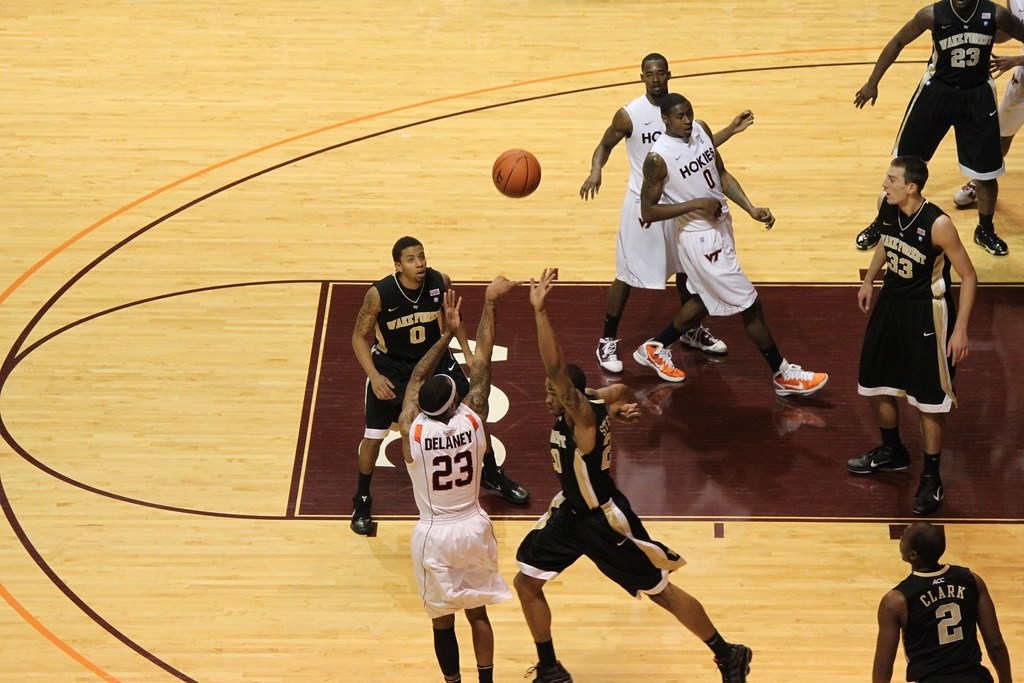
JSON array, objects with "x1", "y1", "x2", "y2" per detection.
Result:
[{"x1": 492, "y1": 149, "x2": 542, "y2": 199}]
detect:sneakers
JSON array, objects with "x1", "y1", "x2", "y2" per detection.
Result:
[
  {"x1": 856, "y1": 218, "x2": 881, "y2": 250},
  {"x1": 775, "y1": 399, "x2": 826, "y2": 436},
  {"x1": 953, "y1": 180, "x2": 977, "y2": 205},
  {"x1": 680, "y1": 325, "x2": 727, "y2": 354},
  {"x1": 595, "y1": 337, "x2": 624, "y2": 372},
  {"x1": 846, "y1": 444, "x2": 911, "y2": 474},
  {"x1": 524, "y1": 660, "x2": 573, "y2": 683},
  {"x1": 480, "y1": 465, "x2": 530, "y2": 502},
  {"x1": 714, "y1": 644, "x2": 752, "y2": 683},
  {"x1": 974, "y1": 224, "x2": 1008, "y2": 256},
  {"x1": 634, "y1": 383, "x2": 683, "y2": 415},
  {"x1": 772, "y1": 357, "x2": 829, "y2": 396},
  {"x1": 632, "y1": 337, "x2": 686, "y2": 381},
  {"x1": 912, "y1": 474, "x2": 945, "y2": 514},
  {"x1": 351, "y1": 495, "x2": 373, "y2": 534}
]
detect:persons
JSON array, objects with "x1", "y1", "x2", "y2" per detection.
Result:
[
  {"x1": 349, "y1": 237, "x2": 533, "y2": 533},
  {"x1": 632, "y1": 93, "x2": 829, "y2": 396},
  {"x1": 399, "y1": 276, "x2": 521, "y2": 683},
  {"x1": 872, "y1": 522, "x2": 1013, "y2": 683},
  {"x1": 579, "y1": 53, "x2": 755, "y2": 372},
  {"x1": 853, "y1": 0, "x2": 1024, "y2": 255},
  {"x1": 846, "y1": 156, "x2": 978, "y2": 517},
  {"x1": 513, "y1": 267, "x2": 753, "y2": 683},
  {"x1": 952, "y1": 0, "x2": 1024, "y2": 206}
]
